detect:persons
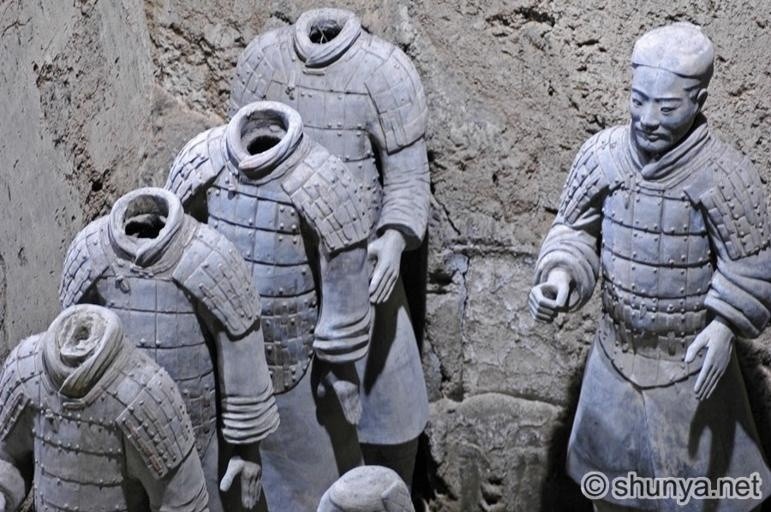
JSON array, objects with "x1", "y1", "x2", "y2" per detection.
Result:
[
  {"x1": 314, "y1": 465, "x2": 416, "y2": 511},
  {"x1": 526, "y1": 18, "x2": 770, "y2": 512},
  {"x1": 161, "y1": 101, "x2": 374, "y2": 511},
  {"x1": 0, "y1": 305, "x2": 209, "y2": 512},
  {"x1": 228, "y1": 6, "x2": 430, "y2": 493},
  {"x1": 56, "y1": 187, "x2": 281, "y2": 512}
]
